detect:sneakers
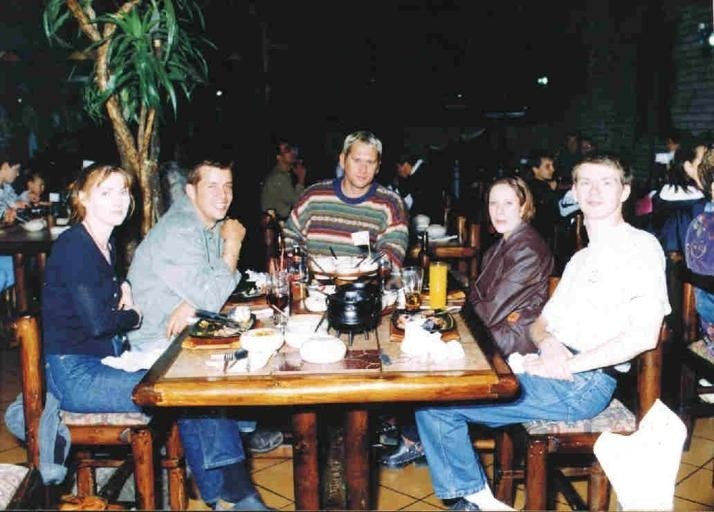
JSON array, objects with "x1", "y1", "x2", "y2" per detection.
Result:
[
  {"x1": 241, "y1": 429, "x2": 284, "y2": 454},
  {"x1": 449, "y1": 496, "x2": 480, "y2": 511},
  {"x1": 378, "y1": 437, "x2": 427, "y2": 469},
  {"x1": 212, "y1": 490, "x2": 272, "y2": 512}
]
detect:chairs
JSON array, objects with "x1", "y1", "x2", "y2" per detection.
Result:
[
  {"x1": 264, "y1": 211, "x2": 288, "y2": 268},
  {"x1": 442, "y1": 206, "x2": 480, "y2": 281},
  {"x1": 573, "y1": 214, "x2": 584, "y2": 251},
  {"x1": 669, "y1": 251, "x2": 714, "y2": 453},
  {"x1": 474, "y1": 277, "x2": 662, "y2": 512},
  {"x1": 14, "y1": 313, "x2": 186, "y2": 512}
]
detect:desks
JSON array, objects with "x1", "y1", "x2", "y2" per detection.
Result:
[{"x1": 2, "y1": 219, "x2": 70, "y2": 319}]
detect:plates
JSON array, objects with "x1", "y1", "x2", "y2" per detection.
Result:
[{"x1": 191, "y1": 209, "x2": 459, "y2": 364}]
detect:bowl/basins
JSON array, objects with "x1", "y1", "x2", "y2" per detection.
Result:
[{"x1": 24, "y1": 221, "x2": 44, "y2": 231}]
